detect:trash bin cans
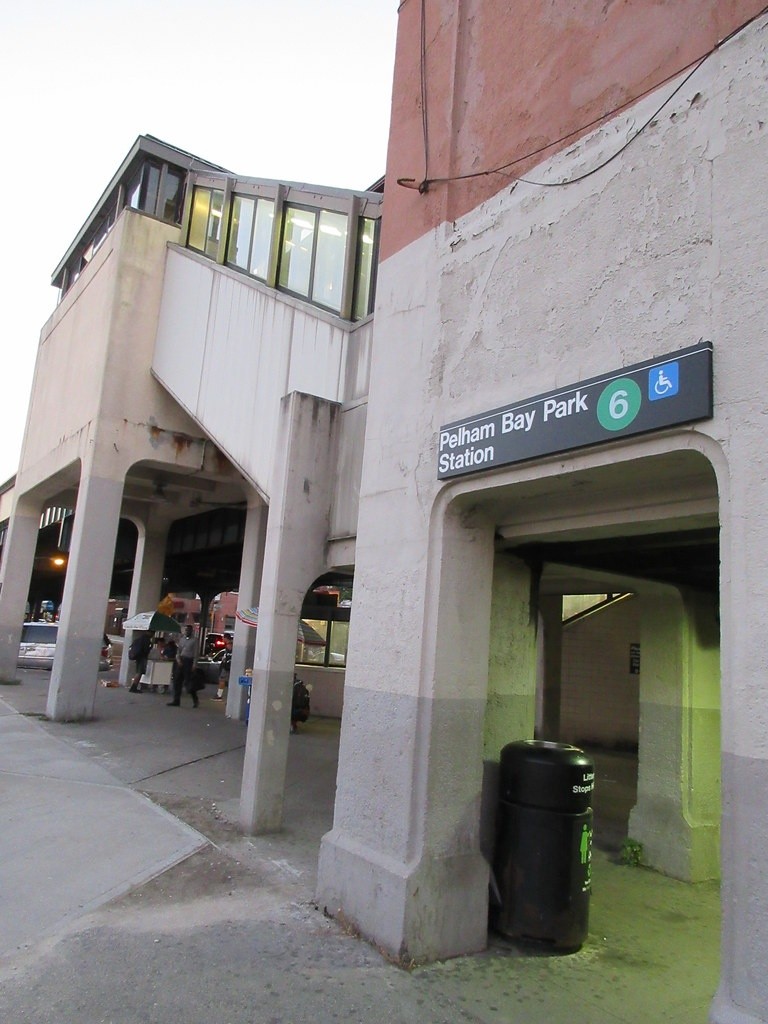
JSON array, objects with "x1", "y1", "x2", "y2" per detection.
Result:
[{"x1": 499, "y1": 740, "x2": 593, "y2": 952}]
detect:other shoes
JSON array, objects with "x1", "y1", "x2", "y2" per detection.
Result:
[
  {"x1": 129, "y1": 687, "x2": 133, "y2": 692},
  {"x1": 167, "y1": 701, "x2": 180, "y2": 706},
  {"x1": 210, "y1": 695, "x2": 222, "y2": 702},
  {"x1": 132, "y1": 688, "x2": 143, "y2": 693},
  {"x1": 193, "y1": 701, "x2": 199, "y2": 708}
]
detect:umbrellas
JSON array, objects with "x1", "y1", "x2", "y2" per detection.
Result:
[
  {"x1": 121, "y1": 610, "x2": 184, "y2": 633},
  {"x1": 234, "y1": 607, "x2": 326, "y2": 647}
]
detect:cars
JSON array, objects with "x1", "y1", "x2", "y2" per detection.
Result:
[{"x1": 16, "y1": 622, "x2": 114, "y2": 672}]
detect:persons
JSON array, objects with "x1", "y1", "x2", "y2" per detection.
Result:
[
  {"x1": 125, "y1": 630, "x2": 156, "y2": 695},
  {"x1": 166, "y1": 623, "x2": 201, "y2": 710},
  {"x1": 211, "y1": 633, "x2": 233, "y2": 700}
]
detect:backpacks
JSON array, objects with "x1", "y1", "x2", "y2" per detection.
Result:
[
  {"x1": 129, "y1": 637, "x2": 151, "y2": 660},
  {"x1": 222, "y1": 647, "x2": 232, "y2": 671},
  {"x1": 291, "y1": 673, "x2": 310, "y2": 722}
]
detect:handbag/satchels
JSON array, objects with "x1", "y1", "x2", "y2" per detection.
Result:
[{"x1": 184, "y1": 670, "x2": 205, "y2": 694}]
